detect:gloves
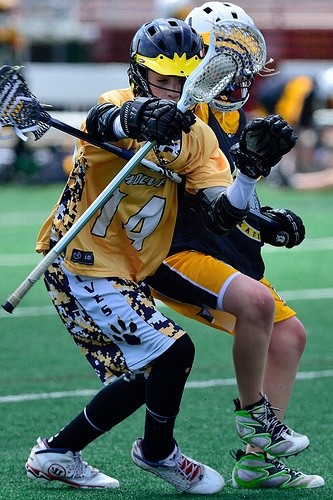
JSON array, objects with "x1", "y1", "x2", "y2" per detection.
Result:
[
  {"x1": 120, "y1": 97, "x2": 196, "y2": 146},
  {"x1": 259, "y1": 206, "x2": 306, "y2": 248},
  {"x1": 229, "y1": 114, "x2": 298, "y2": 179}
]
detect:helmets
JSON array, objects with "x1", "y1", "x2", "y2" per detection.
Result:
[
  {"x1": 126, "y1": 18, "x2": 205, "y2": 113},
  {"x1": 184, "y1": 1, "x2": 267, "y2": 111}
]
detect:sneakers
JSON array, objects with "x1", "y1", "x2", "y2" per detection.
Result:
[
  {"x1": 233, "y1": 394, "x2": 311, "y2": 458},
  {"x1": 25, "y1": 437, "x2": 120, "y2": 488},
  {"x1": 230, "y1": 448, "x2": 325, "y2": 489},
  {"x1": 131, "y1": 436, "x2": 226, "y2": 494}
]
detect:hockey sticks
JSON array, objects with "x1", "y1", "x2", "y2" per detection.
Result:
[
  {"x1": 0, "y1": 19, "x2": 268, "y2": 314},
  {"x1": 0, "y1": 64, "x2": 282, "y2": 228}
]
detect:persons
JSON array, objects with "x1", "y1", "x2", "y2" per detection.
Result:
[
  {"x1": 0, "y1": 0, "x2": 92, "y2": 182},
  {"x1": 143, "y1": 2, "x2": 325, "y2": 489},
  {"x1": 249, "y1": 67, "x2": 333, "y2": 191},
  {"x1": 153, "y1": 0, "x2": 195, "y2": 21},
  {"x1": 25, "y1": 16, "x2": 298, "y2": 494}
]
